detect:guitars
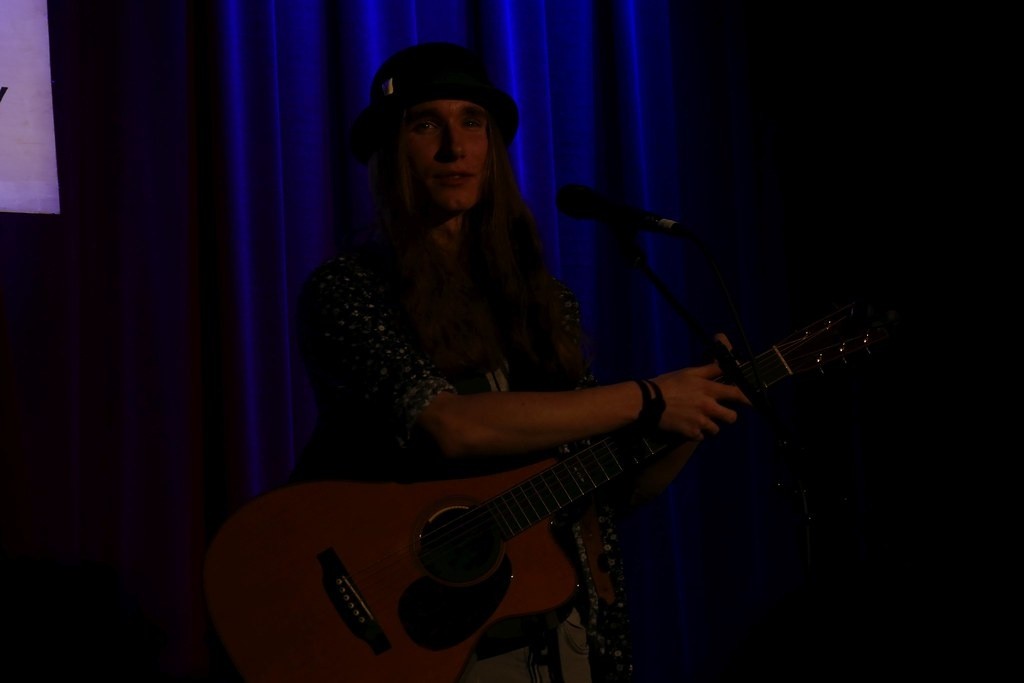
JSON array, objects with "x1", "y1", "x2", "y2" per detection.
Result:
[{"x1": 201, "y1": 305, "x2": 879, "y2": 682}]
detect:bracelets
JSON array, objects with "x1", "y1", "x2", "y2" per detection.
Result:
[{"x1": 638, "y1": 377, "x2": 667, "y2": 435}]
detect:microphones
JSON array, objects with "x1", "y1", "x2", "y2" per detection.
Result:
[{"x1": 555, "y1": 183, "x2": 682, "y2": 240}]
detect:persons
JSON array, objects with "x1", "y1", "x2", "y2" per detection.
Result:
[{"x1": 284, "y1": 43, "x2": 750, "y2": 683}]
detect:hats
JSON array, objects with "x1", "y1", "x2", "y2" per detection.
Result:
[{"x1": 345, "y1": 42, "x2": 519, "y2": 156}]
